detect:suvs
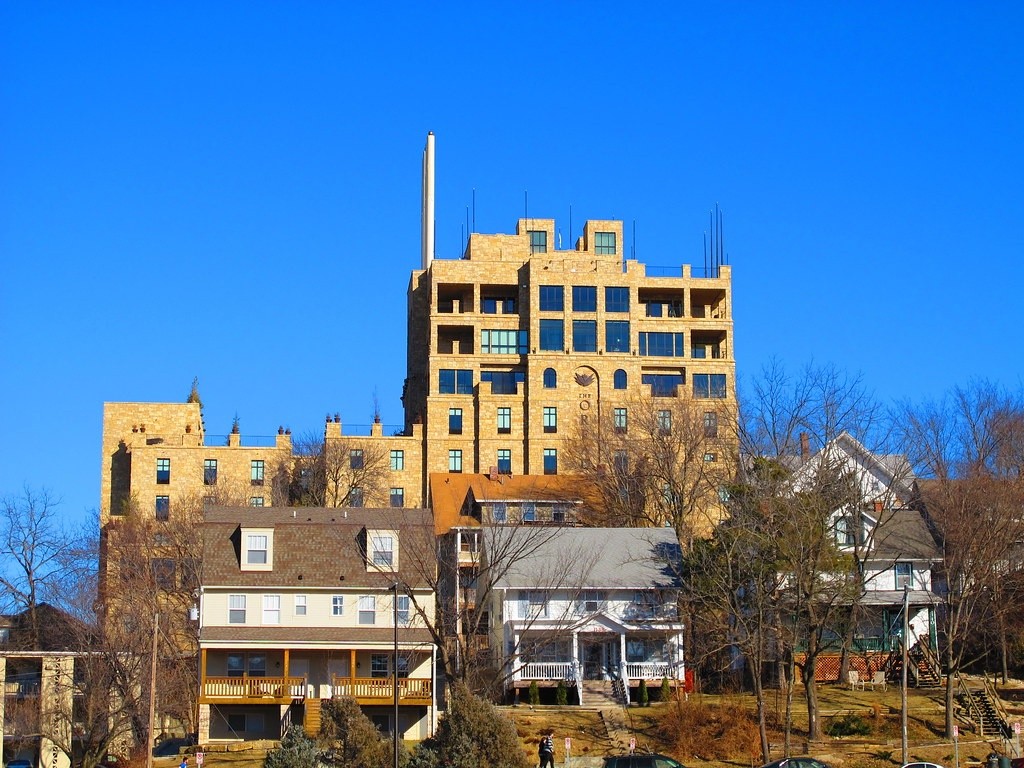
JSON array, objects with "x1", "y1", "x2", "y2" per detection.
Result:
[{"x1": 602, "y1": 753, "x2": 683, "y2": 768}]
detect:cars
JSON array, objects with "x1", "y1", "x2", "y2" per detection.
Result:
[
  {"x1": 5, "y1": 759, "x2": 33, "y2": 768},
  {"x1": 759, "y1": 757, "x2": 830, "y2": 768},
  {"x1": 901, "y1": 761, "x2": 944, "y2": 768}
]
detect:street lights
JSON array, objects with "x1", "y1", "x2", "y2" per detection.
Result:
[{"x1": 386, "y1": 583, "x2": 405, "y2": 768}]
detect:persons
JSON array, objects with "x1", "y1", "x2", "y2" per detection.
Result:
[
  {"x1": 179, "y1": 757, "x2": 188, "y2": 768},
  {"x1": 537, "y1": 730, "x2": 555, "y2": 768}
]
